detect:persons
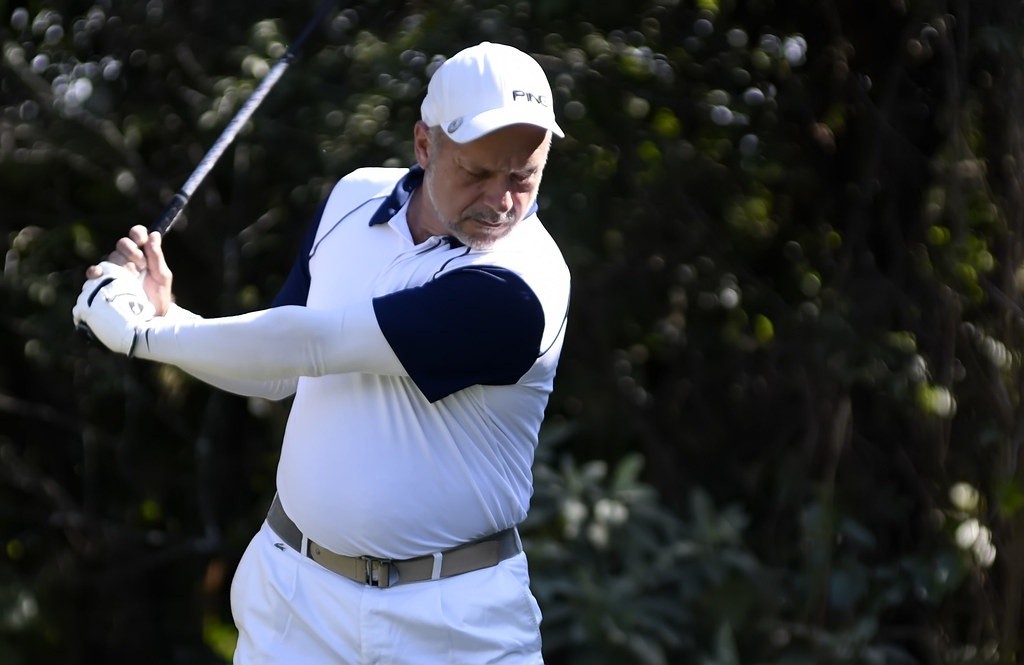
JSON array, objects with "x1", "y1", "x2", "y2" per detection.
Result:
[{"x1": 69, "y1": 40, "x2": 574, "y2": 665}]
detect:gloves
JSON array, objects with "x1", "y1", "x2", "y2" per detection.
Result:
[{"x1": 73, "y1": 262, "x2": 157, "y2": 360}]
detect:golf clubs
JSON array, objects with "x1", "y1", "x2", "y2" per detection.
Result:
[{"x1": 75, "y1": 0, "x2": 326, "y2": 344}]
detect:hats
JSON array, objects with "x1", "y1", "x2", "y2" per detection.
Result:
[{"x1": 421, "y1": 41, "x2": 565, "y2": 145}]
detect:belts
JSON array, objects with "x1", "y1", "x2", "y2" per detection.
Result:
[{"x1": 264, "y1": 491, "x2": 525, "y2": 589}]
